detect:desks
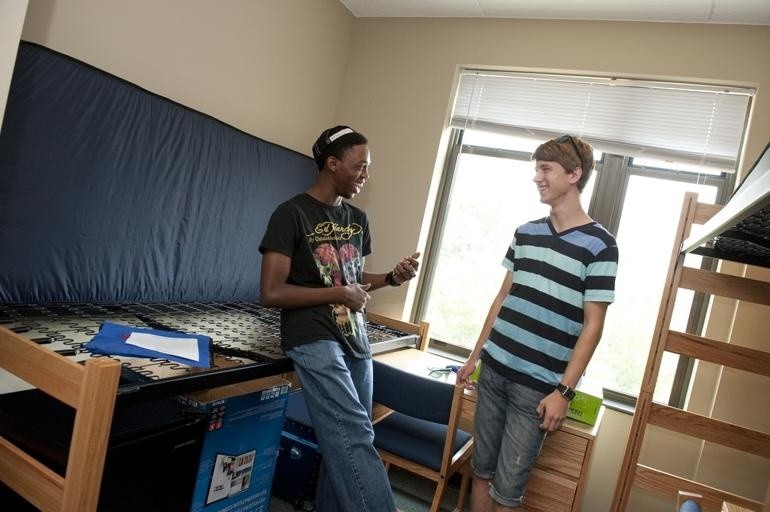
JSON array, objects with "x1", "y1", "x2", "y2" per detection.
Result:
[{"x1": 329, "y1": 344, "x2": 609, "y2": 511}]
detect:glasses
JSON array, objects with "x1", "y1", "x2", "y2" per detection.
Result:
[{"x1": 558, "y1": 135, "x2": 581, "y2": 159}]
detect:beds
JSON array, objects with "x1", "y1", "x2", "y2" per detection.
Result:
[
  {"x1": 609, "y1": 139, "x2": 770, "y2": 510},
  {"x1": 2, "y1": 296, "x2": 434, "y2": 512}
]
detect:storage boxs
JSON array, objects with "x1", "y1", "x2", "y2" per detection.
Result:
[
  {"x1": 563, "y1": 373, "x2": 606, "y2": 427},
  {"x1": 100, "y1": 351, "x2": 292, "y2": 511}
]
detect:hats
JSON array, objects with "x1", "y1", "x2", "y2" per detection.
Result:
[{"x1": 313, "y1": 126, "x2": 355, "y2": 159}]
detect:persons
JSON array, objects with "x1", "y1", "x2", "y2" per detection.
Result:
[
  {"x1": 258, "y1": 125, "x2": 421, "y2": 512},
  {"x1": 456, "y1": 135, "x2": 617, "y2": 512}
]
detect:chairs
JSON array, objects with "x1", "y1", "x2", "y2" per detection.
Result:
[{"x1": 362, "y1": 354, "x2": 480, "y2": 512}]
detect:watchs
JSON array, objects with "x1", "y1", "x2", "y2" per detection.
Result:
[{"x1": 557, "y1": 384, "x2": 576, "y2": 401}]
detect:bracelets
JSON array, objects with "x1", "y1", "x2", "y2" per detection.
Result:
[{"x1": 386, "y1": 270, "x2": 401, "y2": 287}]
